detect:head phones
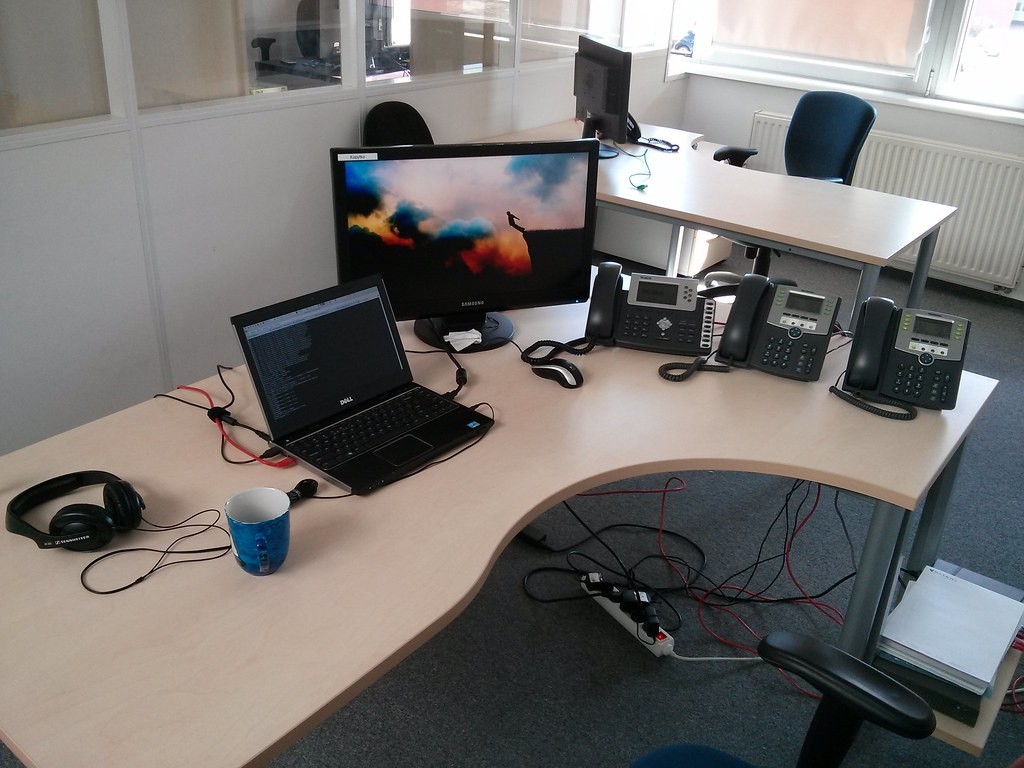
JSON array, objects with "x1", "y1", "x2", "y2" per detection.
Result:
[{"x1": 5, "y1": 470, "x2": 146, "y2": 552}]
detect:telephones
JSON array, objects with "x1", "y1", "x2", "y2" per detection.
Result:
[
  {"x1": 713, "y1": 272, "x2": 843, "y2": 383},
  {"x1": 828, "y1": 295, "x2": 972, "y2": 421},
  {"x1": 597, "y1": 111, "x2": 642, "y2": 143},
  {"x1": 583, "y1": 261, "x2": 716, "y2": 358}
]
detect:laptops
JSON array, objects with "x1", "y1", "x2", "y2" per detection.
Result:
[{"x1": 230, "y1": 274, "x2": 495, "y2": 495}]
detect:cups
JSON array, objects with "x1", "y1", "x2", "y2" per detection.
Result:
[{"x1": 225, "y1": 488, "x2": 290, "y2": 576}]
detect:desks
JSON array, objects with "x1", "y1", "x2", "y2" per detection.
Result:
[
  {"x1": 0, "y1": 264, "x2": 1000, "y2": 766},
  {"x1": 460, "y1": 113, "x2": 961, "y2": 338},
  {"x1": 255, "y1": 56, "x2": 340, "y2": 83}
]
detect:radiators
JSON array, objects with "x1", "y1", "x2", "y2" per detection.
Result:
[{"x1": 747, "y1": 109, "x2": 1024, "y2": 295}]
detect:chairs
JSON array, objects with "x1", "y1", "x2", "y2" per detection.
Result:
[
  {"x1": 704, "y1": 92, "x2": 876, "y2": 290},
  {"x1": 361, "y1": 101, "x2": 439, "y2": 146},
  {"x1": 624, "y1": 629, "x2": 939, "y2": 767},
  {"x1": 251, "y1": 0, "x2": 339, "y2": 75}
]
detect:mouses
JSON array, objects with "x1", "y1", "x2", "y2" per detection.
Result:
[{"x1": 531, "y1": 358, "x2": 583, "y2": 389}]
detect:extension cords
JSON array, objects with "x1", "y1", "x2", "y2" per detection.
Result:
[{"x1": 579, "y1": 576, "x2": 674, "y2": 657}]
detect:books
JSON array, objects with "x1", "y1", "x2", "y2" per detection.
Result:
[{"x1": 876, "y1": 560, "x2": 1024, "y2": 697}]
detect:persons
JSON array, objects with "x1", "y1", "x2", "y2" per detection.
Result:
[{"x1": 506, "y1": 210, "x2": 526, "y2": 234}]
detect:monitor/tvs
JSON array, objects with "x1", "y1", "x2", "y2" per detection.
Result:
[
  {"x1": 572, "y1": 33, "x2": 632, "y2": 159},
  {"x1": 329, "y1": 137, "x2": 600, "y2": 354}
]
